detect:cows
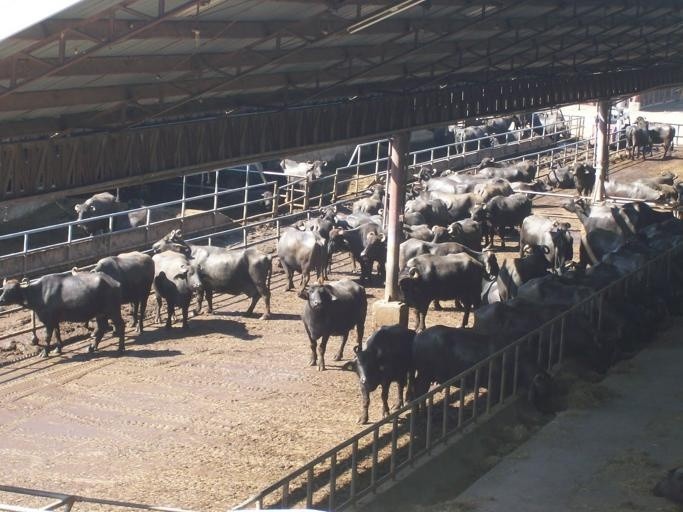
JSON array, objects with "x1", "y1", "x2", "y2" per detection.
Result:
[
  {"x1": 74, "y1": 191, "x2": 124, "y2": 236},
  {"x1": 277, "y1": 156, "x2": 682, "y2": 425},
  {"x1": 608, "y1": 117, "x2": 676, "y2": 161},
  {"x1": 72, "y1": 251, "x2": 156, "y2": 352},
  {"x1": 151, "y1": 250, "x2": 205, "y2": 329},
  {"x1": 152, "y1": 228, "x2": 272, "y2": 320},
  {"x1": 443, "y1": 113, "x2": 572, "y2": 154},
  {"x1": 0, "y1": 272, "x2": 125, "y2": 360}
]
